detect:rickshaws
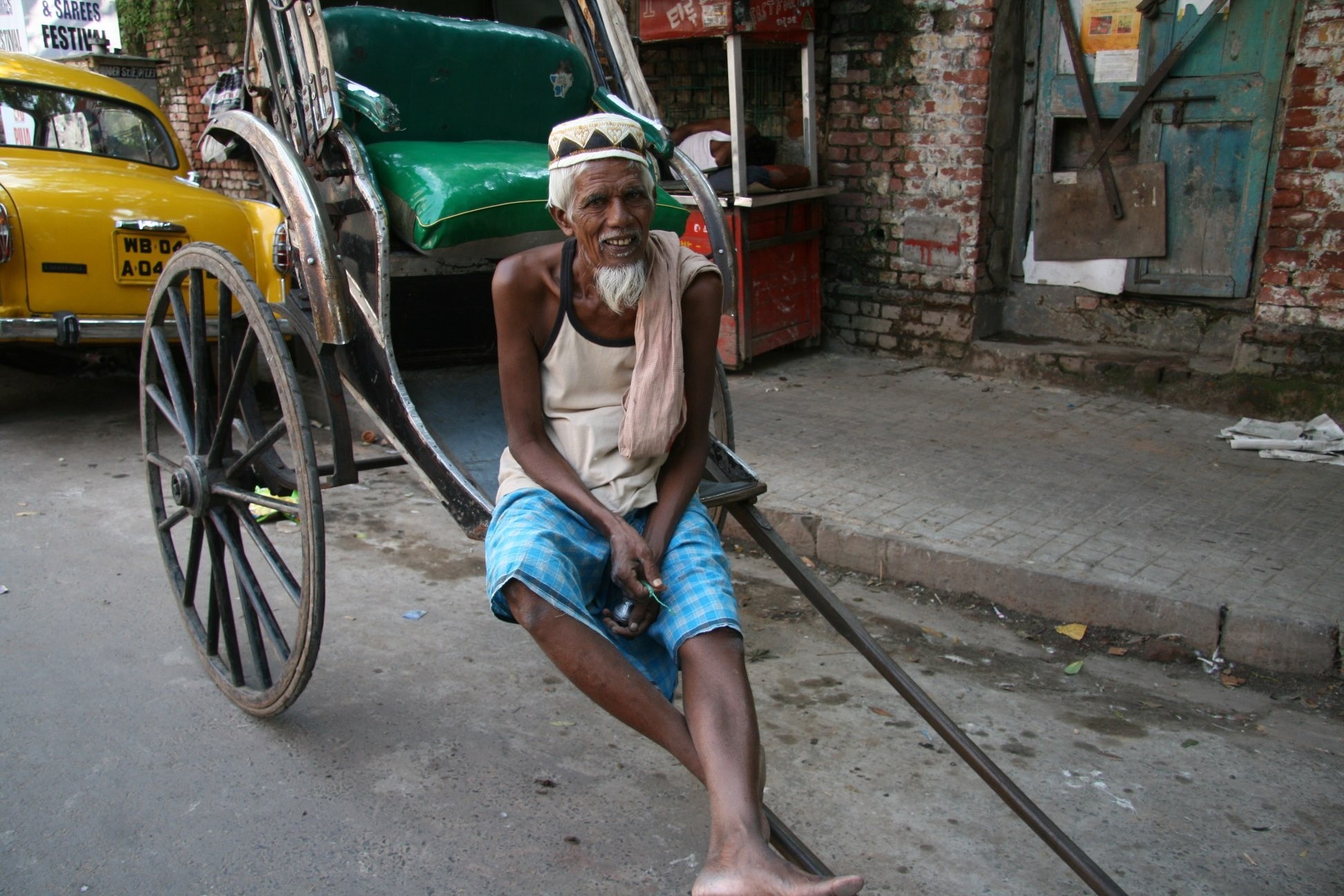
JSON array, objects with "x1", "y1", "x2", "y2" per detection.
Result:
[{"x1": 136, "y1": 0, "x2": 1135, "y2": 896}]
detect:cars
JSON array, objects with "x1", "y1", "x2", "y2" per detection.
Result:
[{"x1": 1, "y1": 50, "x2": 297, "y2": 392}]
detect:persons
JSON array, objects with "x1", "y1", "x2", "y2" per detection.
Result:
[
  {"x1": 484, "y1": 114, "x2": 865, "y2": 896},
  {"x1": 659, "y1": 116, "x2": 779, "y2": 183}
]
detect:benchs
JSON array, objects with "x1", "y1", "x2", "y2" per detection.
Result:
[{"x1": 321, "y1": 5, "x2": 689, "y2": 260}]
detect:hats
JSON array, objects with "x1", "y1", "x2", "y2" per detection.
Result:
[{"x1": 548, "y1": 113, "x2": 650, "y2": 171}]
